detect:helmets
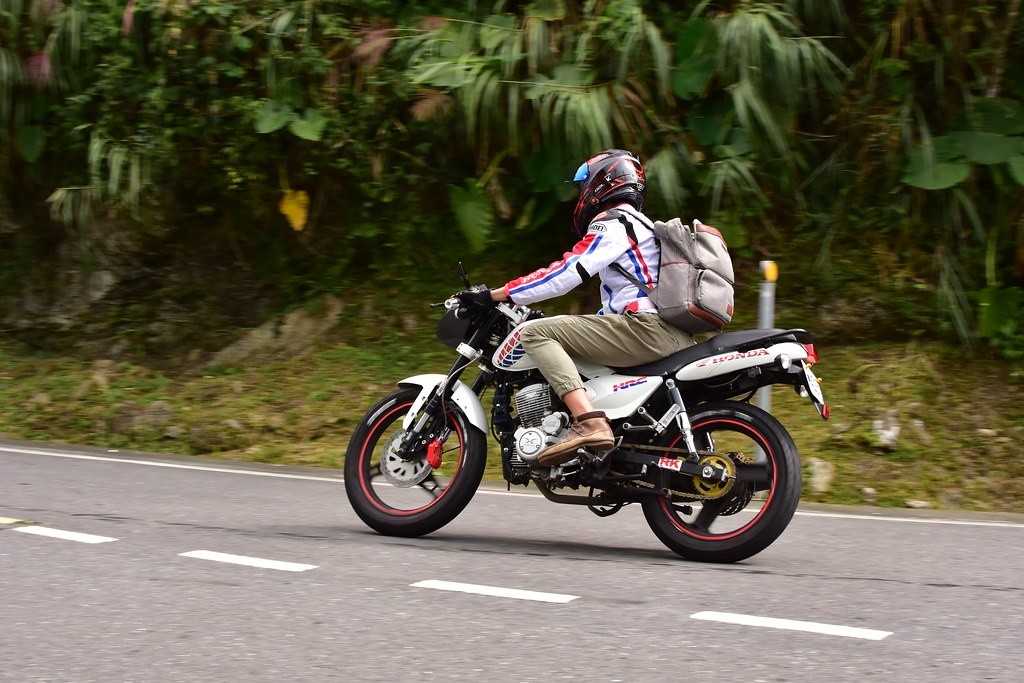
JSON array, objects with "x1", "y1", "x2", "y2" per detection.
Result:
[{"x1": 573, "y1": 149, "x2": 646, "y2": 235}]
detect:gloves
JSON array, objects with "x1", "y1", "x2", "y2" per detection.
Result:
[{"x1": 450, "y1": 288, "x2": 496, "y2": 320}]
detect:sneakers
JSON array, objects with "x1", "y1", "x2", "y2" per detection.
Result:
[{"x1": 537, "y1": 411, "x2": 615, "y2": 466}]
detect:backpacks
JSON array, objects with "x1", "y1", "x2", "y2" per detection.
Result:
[{"x1": 607, "y1": 207, "x2": 735, "y2": 332}]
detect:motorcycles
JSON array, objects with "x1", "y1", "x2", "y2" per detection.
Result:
[{"x1": 343, "y1": 260, "x2": 829, "y2": 564}]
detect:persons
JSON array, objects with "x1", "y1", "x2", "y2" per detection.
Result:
[{"x1": 446, "y1": 149, "x2": 696, "y2": 468}]
